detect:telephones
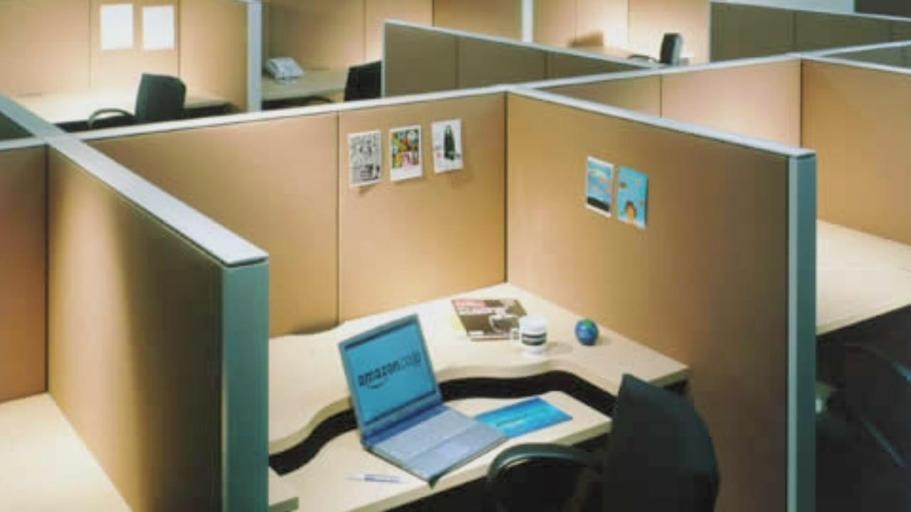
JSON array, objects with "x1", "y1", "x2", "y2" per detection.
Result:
[{"x1": 265, "y1": 56, "x2": 303, "y2": 80}]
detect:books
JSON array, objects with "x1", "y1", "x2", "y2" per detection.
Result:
[{"x1": 450, "y1": 296, "x2": 527, "y2": 341}]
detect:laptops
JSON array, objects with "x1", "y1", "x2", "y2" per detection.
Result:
[{"x1": 336, "y1": 307, "x2": 505, "y2": 483}]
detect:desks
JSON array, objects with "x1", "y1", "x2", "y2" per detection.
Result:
[{"x1": 1, "y1": 43, "x2": 911, "y2": 512}]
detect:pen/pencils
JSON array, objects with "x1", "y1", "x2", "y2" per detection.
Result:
[{"x1": 345, "y1": 474, "x2": 409, "y2": 483}]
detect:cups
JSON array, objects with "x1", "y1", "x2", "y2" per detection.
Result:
[{"x1": 509, "y1": 316, "x2": 548, "y2": 346}]
